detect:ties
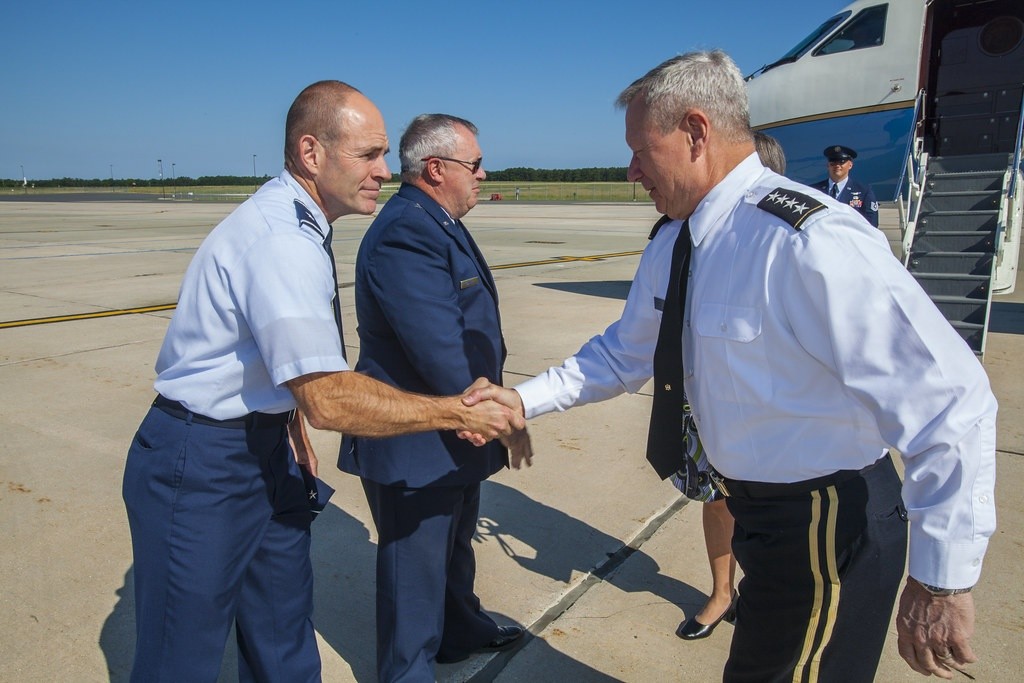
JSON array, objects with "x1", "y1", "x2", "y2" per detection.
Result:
[
  {"x1": 322, "y1": 223, "x2": 348, "y2": 368},
  {"x1": 646, "y1": 218, "x2": 691, "y2": 482}
]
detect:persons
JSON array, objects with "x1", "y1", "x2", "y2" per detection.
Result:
[
  {"x1": 120, "y1": 73, "x2": 544, "y2": 683},
  {"x1": 447, "y1": 49, "x2": 999, "y2": 683},
  {"x1": 334, "y1": 110, "x2": 532, "y2": 683}
]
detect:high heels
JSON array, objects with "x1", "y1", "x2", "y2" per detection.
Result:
[{"x1": 679, "y1": 589, "x2": 739, "y2": 640}]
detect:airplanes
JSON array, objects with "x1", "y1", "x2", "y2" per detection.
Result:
[{"x1": 722, "y1": 0, "x2": 1024, "y2": 354}]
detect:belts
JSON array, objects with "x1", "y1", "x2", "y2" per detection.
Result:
[
  {"x1": 156, "y1": 394, "x2": 295, "y2": 430},
  {"x1": 709, "y1": 454, "x2": 888, "y2": 501}
]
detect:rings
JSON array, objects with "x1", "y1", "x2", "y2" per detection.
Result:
[{"x1": 935, "y1": 651, "x2": 953, "y2": 661}]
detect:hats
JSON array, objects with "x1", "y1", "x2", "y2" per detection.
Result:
[{"x1": 824, "y1": 146, "x2": 857, "y2": 162}]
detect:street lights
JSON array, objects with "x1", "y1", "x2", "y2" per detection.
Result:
[
  {"x1": 172, "y1": 163, "x2": 176, "y2": 194},
  {"x1": 254, "y1": 154, "x2": 257, "y2": 193},
  {"x1": 157, "y1": 159, "x2": 165, "y2": 197}
]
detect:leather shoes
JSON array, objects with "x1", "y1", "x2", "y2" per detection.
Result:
[{"x1": 435, "y1": 625, "x2": 522, "y2": 664}]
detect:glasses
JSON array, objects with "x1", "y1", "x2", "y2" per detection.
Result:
[
  {"x1": 420, "y1": 156, "x2": 483, "y2": 175},
  {"x1": 828, "y1": 160, "x2": 849, "y2": 165}
]
